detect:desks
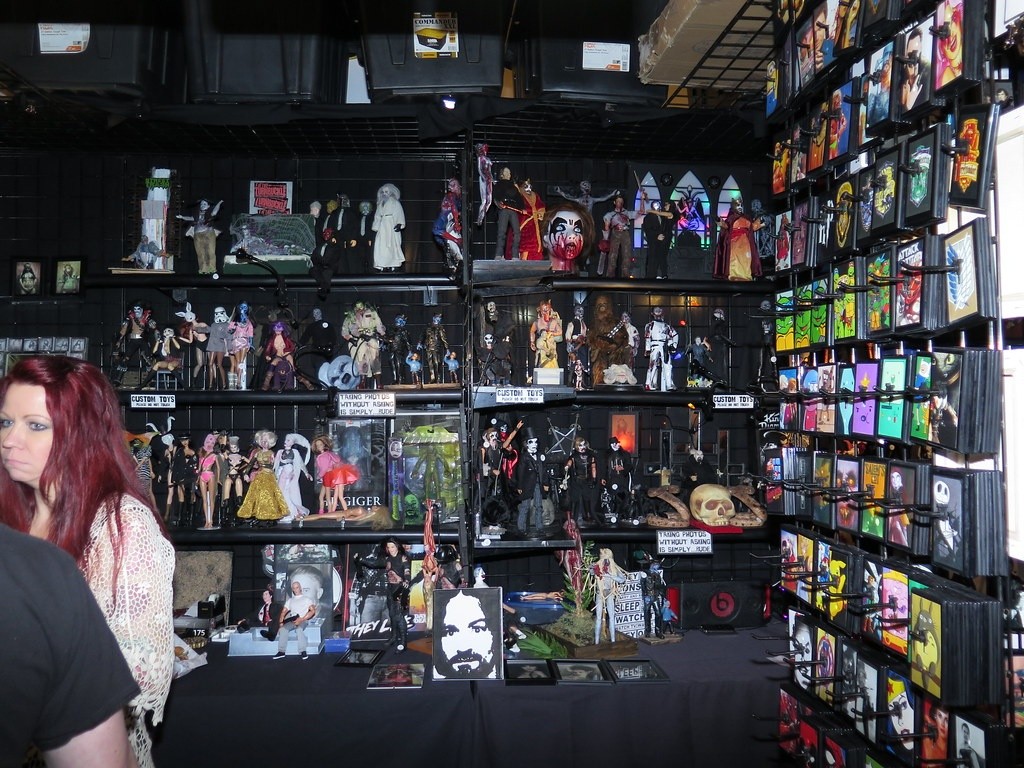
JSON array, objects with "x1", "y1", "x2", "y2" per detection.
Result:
[{"x1": 146, "y1": 624, "x2": 790, "y2": 768}]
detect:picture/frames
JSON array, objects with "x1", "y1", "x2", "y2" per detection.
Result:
[
  {"x1": 335, "y1": 647, "x2": 385, "y2": 668},
  {"x1": 550, "y1": 658, "x2": 615, "y2": 685},
  {"x1": 504, "y1": 656, "x2": 555, "y2": 686},
  {"x1": 602, "y1": 657, "x2": 671, "y2": 684}
]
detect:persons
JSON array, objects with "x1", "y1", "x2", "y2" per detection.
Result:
[
  {"x1": 792, "y1": 616, "x2": 813, "y2": 692},
  {"x1": 288, "y1": 565, "x2": 331, "y2": 623},
  {"x1": 920, "y1": 704, "x2": 981, "y2": 768},
  {"x1": 112, "y1": 164, "x2": 777, "y2": 660},
  {"x1": 515, "y1": 663, "x2": 603, "y2": 681},
  {"x1": 901, "y1": 31, "x2": 930, "y2": 111},
  {"x1": 0, "y1": 356, "x2": 178, "y2": 768},
  {"x1": 795, "y1": 7, "x2": 847, "y2": 173}
]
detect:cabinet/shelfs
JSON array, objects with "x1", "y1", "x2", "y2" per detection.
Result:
[
  {"x1": 460, "y1": 276, "x2": 773, "y2": 639},
  {"x1": 47, "y1": 263, "x2": 455, "y2": 643}
]
